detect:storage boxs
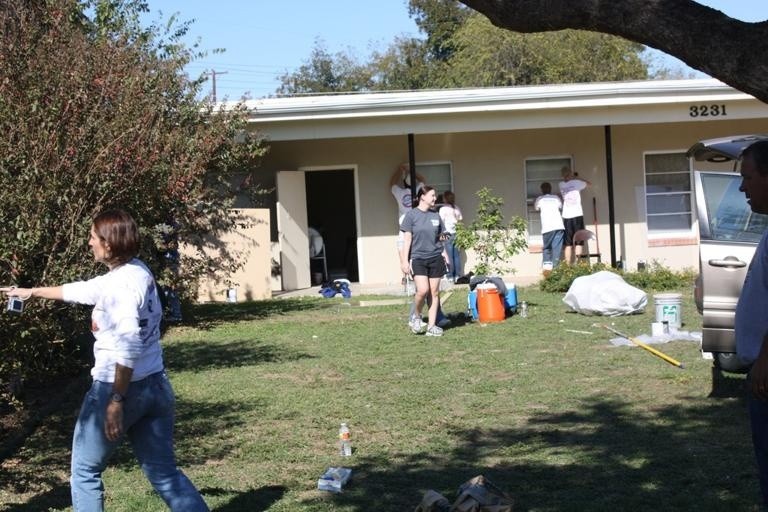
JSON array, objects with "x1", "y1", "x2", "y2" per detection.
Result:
[{"x1": 470, "y1": 282, "x2": 518, "y2": 318}]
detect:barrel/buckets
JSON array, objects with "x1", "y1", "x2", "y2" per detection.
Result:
[
  {"x1": 652, "y1": 293, "x2": 684, "y2": 331},
  {"x1": 467, "y1": 290, "x2": 479, "y2": 319},
  {"x1": 504, "y1": 282, "x2": 518, "y2": 315}
]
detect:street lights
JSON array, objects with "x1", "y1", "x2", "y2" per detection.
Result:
[{"x1": 204, "y1": 68, "x2": 229, "y2": 105}]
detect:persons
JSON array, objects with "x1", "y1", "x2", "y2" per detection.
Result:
[
  {"x1": 398, "y1": 185, "x2": 448, "y2": 337},
  {"x1": 558, "y1": 166, "x2": 592, "y2": 266},
  {"x1": 438, "y1": 191, "x2": 463, "y2": 282},
  {"x1": 391, "y1": 165, "x2": 426, "y2": 282},
  {"x1": 534, "y1": 182, "x2": 566, "y2": 270},
  {"x1": 0, "y1": 208, "x2": 210, "y2": 511},
  {"x1": 156, "y1": 237, "x2": 185, "y2": 320},
  {"x1": 734, "y1": 139, "x2": 767, "y2": 511}
]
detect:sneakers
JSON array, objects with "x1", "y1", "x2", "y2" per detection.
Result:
[
  {"x1": 437, "y1": 318, "x2": 452, "y2": 328},
  {"x1": 408, "y1": 317, "x2": 423, "y2": 334},
  {"x1": 425, "y1": 324, "x2": 444, "y2": 337}
]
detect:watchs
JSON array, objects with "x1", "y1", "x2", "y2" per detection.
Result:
[{"x1": 111, "y1": 392, "x2": 127, "y2": 402}]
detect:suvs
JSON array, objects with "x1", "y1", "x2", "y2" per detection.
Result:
[{"x1": 679, "y1": 130, "x2": 767, "y2": 378}]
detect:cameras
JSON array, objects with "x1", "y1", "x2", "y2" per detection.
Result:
[{"x1": 8, "y1": 297, "x2": 23, "y2": 313}]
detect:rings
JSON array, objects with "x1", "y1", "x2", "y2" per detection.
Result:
[{"x1": 759, "y1": 386, "x2": 764, "y2": 390}]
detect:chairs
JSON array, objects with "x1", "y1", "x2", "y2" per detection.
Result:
[
  {"x1": 311, "y1": 244, "x2": 329, "y2": 281},
  {"x1": 572, "y1": 230, "x2": 600, "y2": 264}
]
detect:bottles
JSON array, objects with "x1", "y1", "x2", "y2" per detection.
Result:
[
  {"x1": 338, "y1": 422, "x2": 352, "y2": 456},
  {"x1": 661, "y1": 320, "x2": 669, "y2": 334},
  {"x1": 229, "y1": 287, "x2": 237, "y2": 303},
  {"x1": 520, "y1": 300, "x2": 527, "y2": 318}
]
